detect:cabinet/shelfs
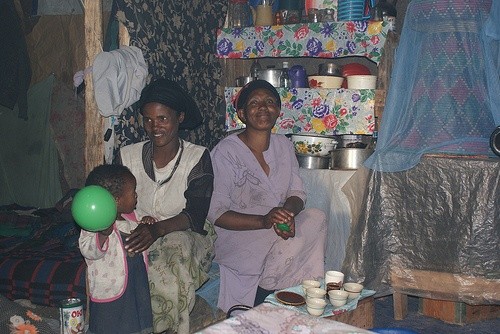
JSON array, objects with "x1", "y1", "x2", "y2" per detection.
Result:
[{"x1": 214, "y1": 18, "x2": 395, "y2": 177}]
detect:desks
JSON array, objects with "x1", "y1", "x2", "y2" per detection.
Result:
[
  {"x1": 272, "y1": 277, "x2": 374, "y2": 332},
  {"x1": 373, "y1": 151, "x2": 500, "y2": 324}
]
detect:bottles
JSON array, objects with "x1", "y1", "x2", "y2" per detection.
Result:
[
  {"x1": 279, "y1": 62, "x2": 291, "y2": 89},
  {"x1": 250, "y1": 58, "x2": 261, "y2": 79},
  {"x1": 226, "y1": 0, "x2": 250, "y2": 27}
]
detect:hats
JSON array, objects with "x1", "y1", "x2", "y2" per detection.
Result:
[{"x1": 139, "y1": 78, "x2": 204, "y2": 130}]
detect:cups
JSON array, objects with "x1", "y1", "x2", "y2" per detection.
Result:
[
  {"x1": 301, "y1": 279, "x2": 321, "y2": 293},
  {"x1": 377, "y1": 0, "x2": 399, "y2": 34},
  {"x1": 328, "y1": 289, "x2": 349, "y2": 308},
  {"x1": 305, "y1": 288, "x2": 327, "y2": 299},
  {"x1": 306, "y1": 297, "x2": 327, "y2": 316},
  {"x1": 344, "y1": 282, "x2": 365, "y2": 298},
  {"x1": 325, "y1": 270, "x2": 344, "y2": 287}
]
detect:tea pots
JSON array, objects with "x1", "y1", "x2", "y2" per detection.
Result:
[{"x1": 249, "y1": 0, "x2": 273, "y2": 26}]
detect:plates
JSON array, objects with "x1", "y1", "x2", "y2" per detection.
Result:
[{"x1": 275, "y1": 291, "x2": 306, "y2": 305}]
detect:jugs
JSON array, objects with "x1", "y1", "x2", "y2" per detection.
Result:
[{"x1": 337, "y1": 0, "x2": 376, "y2": 22}]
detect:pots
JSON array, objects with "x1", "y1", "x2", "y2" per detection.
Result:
[{"x1": 256, "y1": 64, "x2": 284, "y2": 88}]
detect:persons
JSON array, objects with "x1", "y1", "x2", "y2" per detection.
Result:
[
  {"x1": 79, "y1": 164, "x2": 159, "y2": 334},
  {"x1": 114, "y1": 80, "x2": 218, "y2": 334},
  {"x1": 209, "y1": 79, "x2": 329, "y2": 315}
]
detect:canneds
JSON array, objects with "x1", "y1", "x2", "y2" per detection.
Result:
[{"x1": 59, "y1": 298, "x2": 84, "y2": 334}]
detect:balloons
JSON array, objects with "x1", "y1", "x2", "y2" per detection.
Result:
[{"x1": 72, "y1": 186, "x2": 117, "y2": 231}]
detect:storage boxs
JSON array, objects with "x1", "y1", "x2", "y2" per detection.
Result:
[{"x1": 416, "y1": 289, "x2": 500, "y2": 325}]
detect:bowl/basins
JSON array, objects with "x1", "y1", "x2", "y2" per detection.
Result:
[
  {"x1": 307, "y1": 62, "x2": 378, "y2": 92},
  {"x1": 235, "y1": 76, "x2": 254, "y2": 87},
  {"x1": 288, "y1": 134, "x2": 374, "y2": 170}
]
